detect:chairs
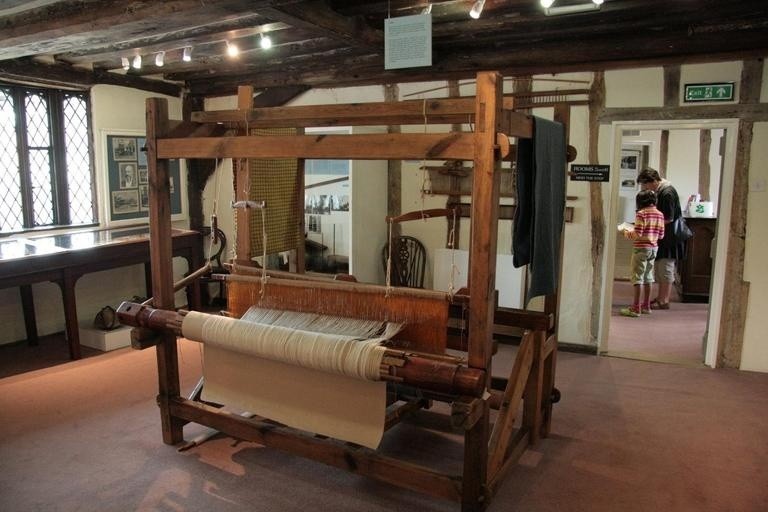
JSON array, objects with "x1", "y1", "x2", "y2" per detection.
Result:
[{"x1": 381, "y1": 236, "x2": 427, "y2": 287}]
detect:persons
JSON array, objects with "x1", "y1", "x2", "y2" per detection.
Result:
[
  {"x1": 121, "y1": 165, "x2": 136, "y2": 187},
  {"x1": 637, "y1": 168, "x2": 682, "y2": 309},
  {"x1": 621, "y1": 189, "x2": 665, "y2": 317}
]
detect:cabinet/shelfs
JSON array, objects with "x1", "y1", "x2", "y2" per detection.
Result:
[{"x1": 674, "y1": 218, "x2": 715, "y2": 303}]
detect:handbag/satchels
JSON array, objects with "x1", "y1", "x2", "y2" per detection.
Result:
[{"x1": 665, "y1": 215, "x2": 693, "y2": 246}]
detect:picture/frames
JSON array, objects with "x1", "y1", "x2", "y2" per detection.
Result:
[{"x1": 100, "y1": 128, "x2": 189, "y2": 226}]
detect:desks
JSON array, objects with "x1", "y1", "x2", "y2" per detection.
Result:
[{"x1": 0, "y1": 224, "x2": 205, "y2": 361}]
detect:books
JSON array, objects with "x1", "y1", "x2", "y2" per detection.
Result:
[{"x1": 618, "y1": 222, "x2": 635, "y2": 232}]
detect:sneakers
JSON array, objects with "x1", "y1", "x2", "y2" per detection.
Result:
[{"x1": 620, "y1": 296, "x2": 670, "y2": 317}]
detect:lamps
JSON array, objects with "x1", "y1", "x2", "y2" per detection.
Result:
[
  {"x1": 115, "y1": 28, "x2": 277, "y2": 71},
  {"x1": 470, "y1": 0, "x2": 486, "y2": 21}
]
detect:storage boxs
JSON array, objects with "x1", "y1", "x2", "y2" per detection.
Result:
[{"x1": 64, "y1": 324, "x2": 135, "y2": 352}]
